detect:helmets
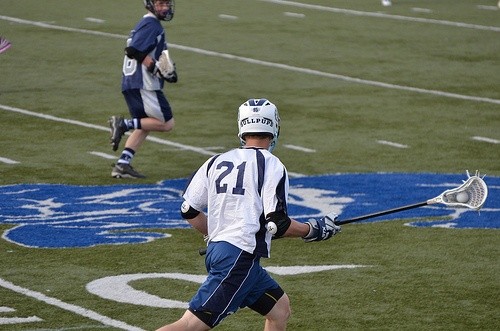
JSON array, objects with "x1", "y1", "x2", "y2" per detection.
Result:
[
  {"x1": 143, "y1": 0, "x2": 175, "y2": 21},
  {"x1": 237, "y1": 98, "x2": 280, "y2": 153}
]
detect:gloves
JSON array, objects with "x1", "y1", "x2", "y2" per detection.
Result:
[
  {"x1": 302, "y1": 212, "x2": 341, "y2": 243},
  {"x1": 147, "y1": 59, "x2": 178, "y2": 83}
]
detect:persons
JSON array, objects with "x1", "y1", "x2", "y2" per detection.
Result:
[
  {"x1": 156, "y1": 98, "x2": 340, "y2": 331},
  {"x1": 108, "y1": 0, "x2": 177, "y2": 180}
]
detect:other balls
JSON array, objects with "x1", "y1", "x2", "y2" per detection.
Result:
[{"x1": 456, "y1": 191, "x2": 469, "y2": 203}]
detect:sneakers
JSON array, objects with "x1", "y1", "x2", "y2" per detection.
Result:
[
  {"x1": 108, "y1": 116, "x2": 128, "y2": 151},
  {"x1": 111, "y1": 163, "x2": 146, "y2": 179}
]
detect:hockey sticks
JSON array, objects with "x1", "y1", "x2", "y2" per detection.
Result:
[
  {"x1": 197, "y1": 176, "x2": 488, "y2": 257},
  {"x1": 155, "y1": 49, "x2": 179, "y2": 83}
]
